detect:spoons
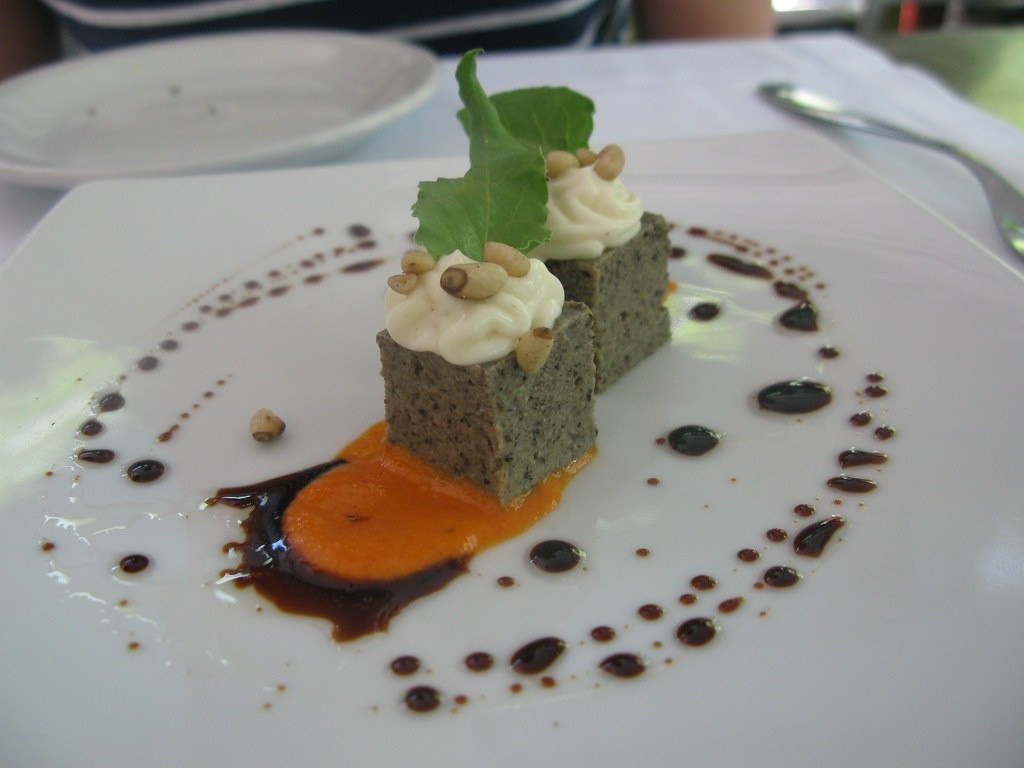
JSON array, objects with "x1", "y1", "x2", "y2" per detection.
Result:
[{"x1": 757, "y1": 84, "x2": 1024, "y2": 257}]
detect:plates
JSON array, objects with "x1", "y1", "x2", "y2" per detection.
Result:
[
  {"x1": 0, "y1": 31, "x2": 441, "y2": 186},
  {"x1": 1, "y1": 130, "x2": 1024, "y2": 768},
  {"x1": 46, "y1": 0, "x2": 599, "y2": 39}
]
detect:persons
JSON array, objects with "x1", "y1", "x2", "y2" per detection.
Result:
[{"x1": 0, "y1": 0, "x2": 776, "y2": 81}]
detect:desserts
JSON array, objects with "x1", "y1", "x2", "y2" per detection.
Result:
[{"x1": 373, "y1": 44, "x2": 672, "y2": 509}]
